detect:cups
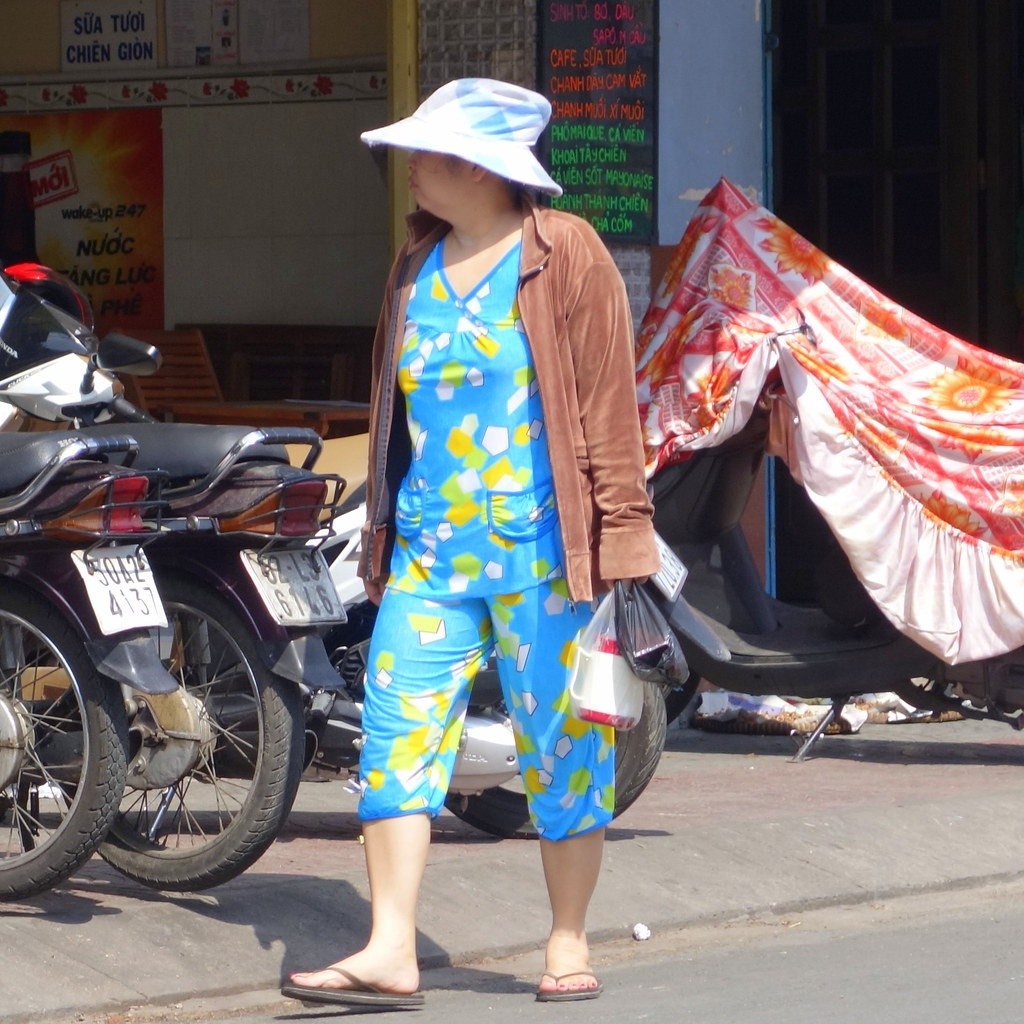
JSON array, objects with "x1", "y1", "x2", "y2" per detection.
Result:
[{"x1": 567, "y1": 637, "x2": 645, "y2": 728}]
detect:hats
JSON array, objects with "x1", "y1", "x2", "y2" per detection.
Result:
[{"x1": 358, "y1": 77, "x2": 564, "y2": 202}]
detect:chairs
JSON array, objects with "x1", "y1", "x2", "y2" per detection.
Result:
[{"x1": 115, "y1": 325, "x2": 222, "y2": 424}]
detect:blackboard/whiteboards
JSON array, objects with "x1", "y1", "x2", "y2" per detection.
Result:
[{"x1": 534, "y1": 0, "x2": 663, "y2": 245}]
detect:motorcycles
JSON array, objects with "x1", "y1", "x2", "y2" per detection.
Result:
[
  {"x1": 0, "y1": 428, "x2": 216, "y2": 903},
  {"x1": 646, "y1": 179, "x2": 1024, "y2": 765},
  {"x1": 309, "y1": 472, "x2": 738, "y2": 842},
  {"x1": 0, "y1": 260, "x2": 350, "y2": 891}
]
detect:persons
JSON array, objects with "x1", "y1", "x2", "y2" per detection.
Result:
[{"x1": 280, "y1": 77, "x2": 660, "y2": 1006}]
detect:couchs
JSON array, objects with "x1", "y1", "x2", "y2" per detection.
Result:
[{"x1": 178, "y1": 320, "x2": 378, "y2": 441}]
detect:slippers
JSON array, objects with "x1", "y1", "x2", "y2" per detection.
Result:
[
  {"x1": 535, "y1": 970, "x2": 604, "y2": 1002},
  {"x1": 282, "y1": 964, "x2": 424, "y2": 1008}
]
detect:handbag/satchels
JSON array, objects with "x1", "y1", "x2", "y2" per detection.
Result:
[
  {"x1": 568, "y1": 590, "x2": 646, "y2": 733},
  {"x1": 613, "y1": 580, "x2": 691, "y2": 690}
]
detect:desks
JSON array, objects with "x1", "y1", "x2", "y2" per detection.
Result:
[{"x1": 177, "y1": 398, "x2": 372, "y2": 438}]
